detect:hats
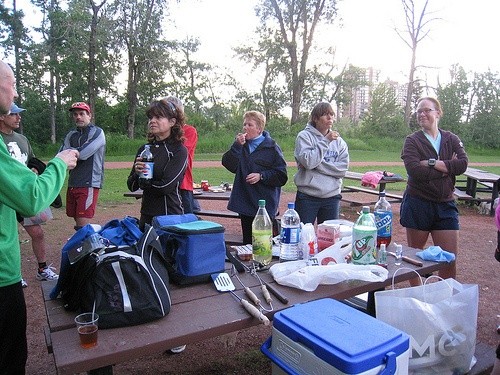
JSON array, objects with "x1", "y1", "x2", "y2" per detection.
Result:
[
  {"x1": 69, "y1": 102, "x2": 91, "y2": 113},
  {"x1": 9, "y1": 102, "x2": 27, "y2": 113}
]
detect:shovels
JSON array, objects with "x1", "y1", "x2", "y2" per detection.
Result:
[{"x1": 211, "y1": 272, "x2": 269, "y2": 325}]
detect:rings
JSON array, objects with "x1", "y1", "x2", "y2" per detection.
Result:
[{"x1": 136, "y1": 165, "x2": 138, "y2": 168}]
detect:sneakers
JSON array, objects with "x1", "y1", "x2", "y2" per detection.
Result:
[
  {"x1": 36, "y1": 263, "x2": 59, "y2": 281},
  {"x1": 21, "y1": 279, "x2": 28, "y2": 288}
]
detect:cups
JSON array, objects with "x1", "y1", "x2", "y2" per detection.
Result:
[
  {"x1": 201, "y1": 180, "x2": 210, "y2": 191},
  {"x1": 74, "y1": 312, "x2": 100, "y2": 347}
]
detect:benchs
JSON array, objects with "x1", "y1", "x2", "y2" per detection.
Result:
[
  {"x1": 454, "y1": 179, "x2": 500, "y2": 207},
  {"x1": 339, "y1": 185, "x2": 403, "y2": 208},
  {"x1": 190, "y1": 207, "x2": 240, "y2": 220}
]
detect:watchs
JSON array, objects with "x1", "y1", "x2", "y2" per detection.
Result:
[
  {"x1": 259, "y1": 172, "x2": 264, "y2": 182},
  {"x1": 428, "y1": 158, "x2": 436, "y2": 169}
]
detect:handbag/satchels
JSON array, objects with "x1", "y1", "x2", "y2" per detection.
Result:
[
  {"x1": 374, "y1": 268, "x2": 478, "y2": 375},
  {"x1": 50, "y1": 213, "x2": 226, "y2": 330}
]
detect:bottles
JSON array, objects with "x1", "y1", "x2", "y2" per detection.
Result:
[
  {"x1": 378, "y1": 244, "x2": 387, "y2": 265},
  {"x1": 351, "y1": 206, "x2": 377, "y2": 265},
  {"x1": 373, "y1": 192, "x2": 392, "y2": 248},
  {"x1": 251, "y1": 199, "x2": 273, "y2": 265},
  {"x1": 139, "y1": 145, "x2": 155, "y2": 190},
  {"x1": 279, "y1": 203, "x2": 300, "y2": 262},
  {"x1": 394, "y1": 245, "x2": 403, "y2": 266}
]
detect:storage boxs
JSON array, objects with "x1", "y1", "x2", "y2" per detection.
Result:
[{"x1": 261, "y1": 298, "x2": 410, "y2": 375}]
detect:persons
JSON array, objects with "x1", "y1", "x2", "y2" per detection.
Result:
[
  {"x1": 162, "y1": 96, "x2": 199, "y2": 215},
  {"x1": 293, "y1": 102, "x2": 349, "y2": 226},
  {"x1": 221, "y1": 110, "x2": 289, "y2": 246},
  {"x1": 57, "y1": 102, "x2": 107, "y2": 227},
  {"x1": 126, "y1": 100, "x2": 189, "y2": 353},
  {"x1": 0, "y1": 103, "x2": 60, "y2": 289},
  {"x1": 0, "y1": 60, "x2": 80, "y2": 375},
  {"x1": 401, "y1": 96, "x2": 469, "y2": 286}
]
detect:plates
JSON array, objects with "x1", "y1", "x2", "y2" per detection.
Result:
[
  {"x1": 193, "y1": 185, "x2": 202, "y2": 194},
  {"x1": 208, "y1": 185, "x2": 233, "y2": 193}
]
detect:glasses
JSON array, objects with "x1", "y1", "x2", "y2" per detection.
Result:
[{"x1": 417, "y1": 108, "x2": 438, "y2": 115}]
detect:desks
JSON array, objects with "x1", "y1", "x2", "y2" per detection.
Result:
[
  {"x1": 461, "y1": 167, "x2": 500, "y2": 204},
  {"x1": 36, "y1": 221, "x2": 456, "y2": 375},
  {"x1": 344, "y1": 172, "x2": 406, "y2": 199},
  {"x1": 122, "y1": 181, "x2": 244, "y2": 243}
]
